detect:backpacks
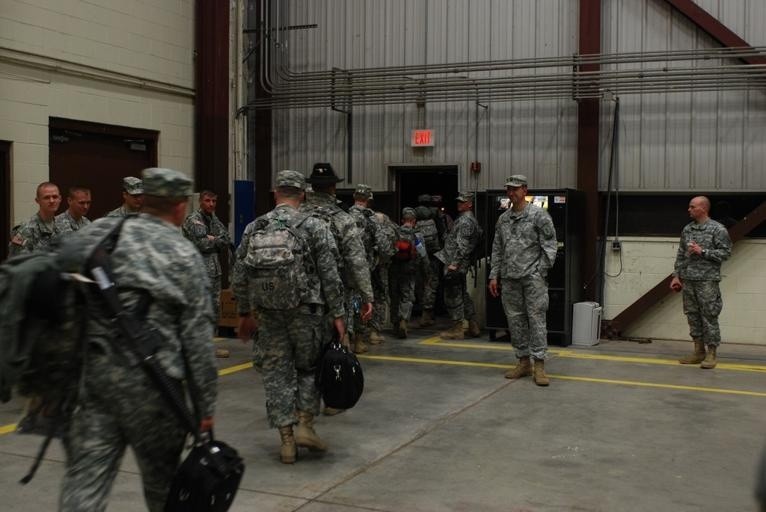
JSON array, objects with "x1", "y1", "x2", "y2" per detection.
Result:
[
  {"x1": 1, "y1": 219, "x2": 122, "y2": 486},
  {"x1": 303, "y1": 205, "x2": 342, "y2": 252},
  {"x1": 416, "y1": 222, "x2": 437, "y2": 249},
  {"x1": 245, "y1": 213, "x2": 318, "y2": 310},
  {"x1": 471, "y1": 227, "x2": 484, "y2": 264}
]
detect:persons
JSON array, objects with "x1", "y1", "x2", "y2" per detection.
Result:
[
  {"x1": 230, "y1": 169, "x2": 346, "y2": 464},
  {"x1": 488, "y1": 175, "x2": 558, "y2": 386},
  {"x1": 669, "y1": 196, "x2": 732, "y2": 368},
  {"x1": 7, "y1": 181, "x2": 66, "y2": 260},
  {"x1": 347, "y1": 192, "x2": 477, "y2": 352},
  {"x1": 56, "y1": 182, "x2": 96, "y2": 247},
  {"x1": 107, "y1": 176, "x2": 144, "y2": 217},
  {"x1": 181, "y1": 190, "x2": 231, "y2": 360},
  {"x1": 298, "y1": 163, "x2": 373, "y2": 415},
  {"x1": 59, "y1": 166, "x2": 218, "y2": 511}
]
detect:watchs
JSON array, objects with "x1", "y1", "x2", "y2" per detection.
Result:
[{"x1": 701, "y1": 249, "x2": 706, "y2": 255}]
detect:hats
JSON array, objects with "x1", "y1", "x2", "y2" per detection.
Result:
[
  {"x1": 274, "y1": 163, "x2": 527, "y2": 216},
  {"x1": 123, "y1": 176, "x2": 144, "y2": 195},
  {"x1": 144, "y1": 167, "x2": 192, "y2": 198}
]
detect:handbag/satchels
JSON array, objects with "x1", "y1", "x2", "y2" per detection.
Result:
[
  {"x1": 439, "y1": 271, "x2": 462, "y2": 289},
  {"x1": 163, "y1": 440, "x2": 244, "y2": 512},
  {"x1": 315, "y1": 337, "x2": 364, "y2": 410}
]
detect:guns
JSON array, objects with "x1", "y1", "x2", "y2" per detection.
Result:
[{"x1": 83, "y1": 248, "x2": 207, "y2": 445}]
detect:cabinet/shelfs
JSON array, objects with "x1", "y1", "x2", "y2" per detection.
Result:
[{"x1": 483, "y1": 189, "x2": 587, "y2": 347}]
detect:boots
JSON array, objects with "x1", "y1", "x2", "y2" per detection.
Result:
[
  {"x1": 701, "y1": 345, "x2": 717, "y2": 369},
  {"x1": 340, "y1": 311, "x2": 480, "y2": 353},
  {"x1": 216, "y1": 349, "x2": 228, "y2": 357},
  {"x1": 505, "y1": 357, "x2": 532, "y2": 378},
  {"x1": 533, "y1": 359, "x2": 549, "y2": 385},
  {"x1": 278, "y1": 424, "x2": 296, "y2": 463},
  {"x1": 680, "y1": 342, "x2": 706, "y2": 364},
  {"x1": 295, "y1": 410, "x2": 326, "y2": 451}
]
